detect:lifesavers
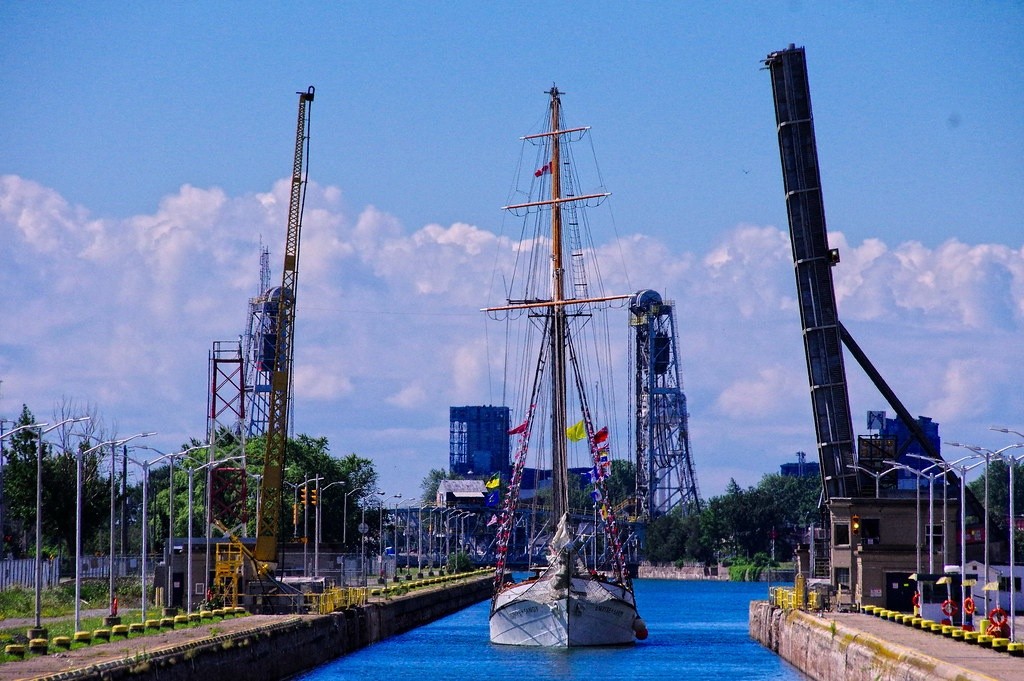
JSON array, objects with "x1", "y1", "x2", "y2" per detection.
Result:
[
  {"x1": 942, "y1": 600, "x2": 959, "y2": 617},
  {"x1": 988, "y1": 608, "x2": 1008, "y2": 627},
  {"x1": 912, "y1": 594, "x2": 921, "y2": 608},
  {"x1": 964, "y1": 598, "x2": 975, "y2": 614}
]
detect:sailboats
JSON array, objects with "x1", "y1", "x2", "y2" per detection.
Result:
[{"x1": 486, "y1": 78, "x2": 653, "y2": 651}]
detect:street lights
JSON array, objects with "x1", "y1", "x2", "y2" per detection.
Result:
[
  {"x1": 0, "y1": 415, "x2": 246, "y2": 635},
  {"x1": 846, "y1": 463, "x2": 905, "y2": 497},
  {"x1": 279, "y1": 473, "x2": 473, "y2": 585},
  {"x1": 882, "y1": 427, "x2": 1024, "y2": 646}
]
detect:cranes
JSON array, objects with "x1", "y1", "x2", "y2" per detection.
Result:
[{"x1": 203, "y1": 84, "x2": 317, "y2": 610}]
